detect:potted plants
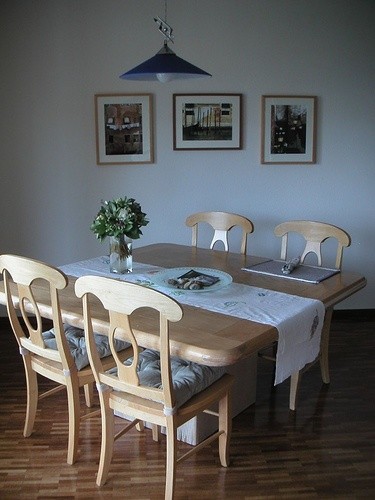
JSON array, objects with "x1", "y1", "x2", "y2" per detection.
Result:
[{"x1": 91, "y1": 196, "x2": 149, "y2": 274}]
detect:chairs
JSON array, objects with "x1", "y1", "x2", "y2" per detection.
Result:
[{"x1": 0, "y1": 211, "x2": 351, "y2": 500}]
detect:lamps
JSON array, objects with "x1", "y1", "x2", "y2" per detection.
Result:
[{"x1": 120, "y1": 0, "x2": 213, "y2": 83}]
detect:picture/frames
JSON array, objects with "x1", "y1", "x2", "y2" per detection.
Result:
[
  {"x1": 172, "y1": 93, "x2": 243, "y2": 150},
  {"x1": 95, "y1": 93, "x2": 155, "y2": 165},
  {"x1": 260, "y1": 94, "x2": 318, "y2": 164}
]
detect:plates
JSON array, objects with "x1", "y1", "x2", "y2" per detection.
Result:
[{"x1": 150, "y1": 266, "x2": 232, "y2": 292}]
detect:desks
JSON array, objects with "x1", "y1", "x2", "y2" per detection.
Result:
[{"x1": 0, "y1": 243, "x2": 367, "y2": 446}]
